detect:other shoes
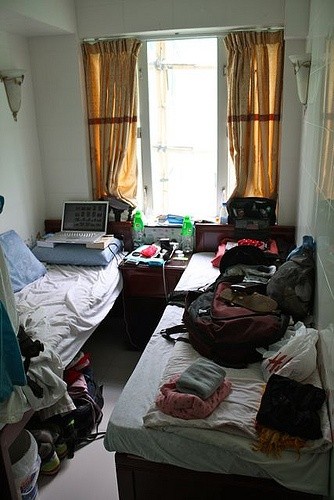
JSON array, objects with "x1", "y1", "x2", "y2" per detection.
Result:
[{"x1": 31, "y1": 428, "x2": 66, "y2": 475}]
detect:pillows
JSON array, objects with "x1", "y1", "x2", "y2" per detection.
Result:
[
  {"x1": 0, "y1": 231, "x2": 48, "y2": 293},
  {"x1": 32, "y1": 238, "x2": 124, "y2": 266}
]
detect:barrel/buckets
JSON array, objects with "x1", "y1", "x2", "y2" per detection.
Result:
[{"x1": 8, "y1": 428, "x2": 40, "y2": 500}]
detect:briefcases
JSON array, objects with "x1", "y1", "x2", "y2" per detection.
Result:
[{"x1": 37, "y1": 371, "x2": 104, "y2": 435}]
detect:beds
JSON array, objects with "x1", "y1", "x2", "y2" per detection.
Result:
[
  {"x1": 0, "y1": 219, "x2": 133, "y2": 499},
  {"x1": 117, "y1": 250, "x2": 192, "y2": 352},
  {"x1": 103, "y1": 221, "x2": 334, "y2": 500}
]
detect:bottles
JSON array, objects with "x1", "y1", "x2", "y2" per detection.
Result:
[
  {"x1": 181, "y1": 215, "x2": 193, "y2": 253},
  {"x1": 133, "y1": 211, "x2": 144, "y2": 241},
  {"x1": 220, "y1": 203, "x2": 228, "y2": 224}
]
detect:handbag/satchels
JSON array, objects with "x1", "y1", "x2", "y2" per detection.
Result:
[
  {"x1": 182, "y1": 283, "x2": 283, "y2": 371},
  {"x1": 261, "y1": 324, "x2": 317, "y2": 385},
  {"x1": 267, "y1": 243, "x2": 314, "y2": 322}
]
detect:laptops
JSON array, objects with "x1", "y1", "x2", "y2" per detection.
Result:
[{"x1": 45, "y1": 201, "x2": 109, "y2": 243}]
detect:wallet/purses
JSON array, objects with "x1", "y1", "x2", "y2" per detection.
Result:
[{"x1": 141, "y1": 246, "x2": 157, "y2": 258}]
